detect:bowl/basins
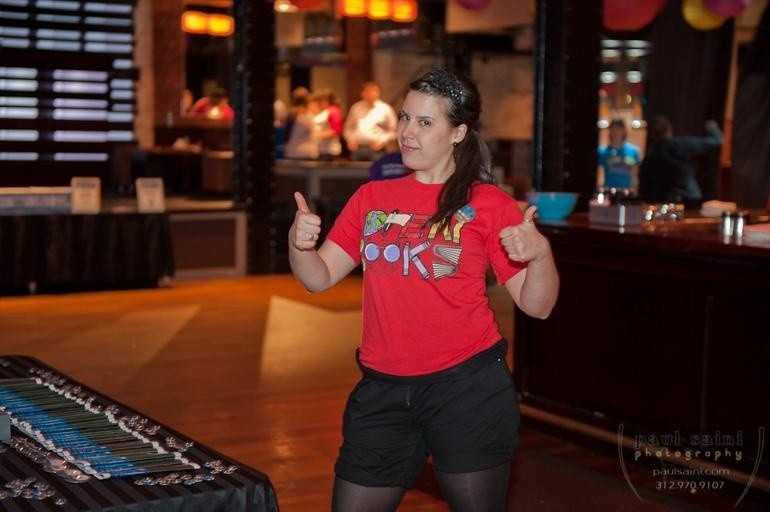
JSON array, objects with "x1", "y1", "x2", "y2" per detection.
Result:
[{"x1": 525, "y1": 192, "x2": 578, "y2": 221}]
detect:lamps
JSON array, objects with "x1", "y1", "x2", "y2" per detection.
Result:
[{"x1": 180, "y1": 1, "x2": 420, "y2": 38}]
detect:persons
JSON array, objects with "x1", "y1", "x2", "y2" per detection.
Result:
[
  {"x1": 286, "y1": 67, "x2": 564, "y2": 509},
  {"x1": 596, "y1": 117, "x2": 643, "y2": 191},
  {"x1": 181, "y1": 77, "x2": 403, "y2": 164},
  {"x1": 637, "y1": 113, "x2": 729, "y2": 210}
]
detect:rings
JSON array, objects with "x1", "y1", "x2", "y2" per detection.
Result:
[{"x1": 308, "y1": 232, "x2": 315, "y2": 241}]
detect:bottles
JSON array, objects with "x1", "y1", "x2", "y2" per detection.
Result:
[{"x1": 721, "y1": 210, "x2": 748, "y2": 238}]
